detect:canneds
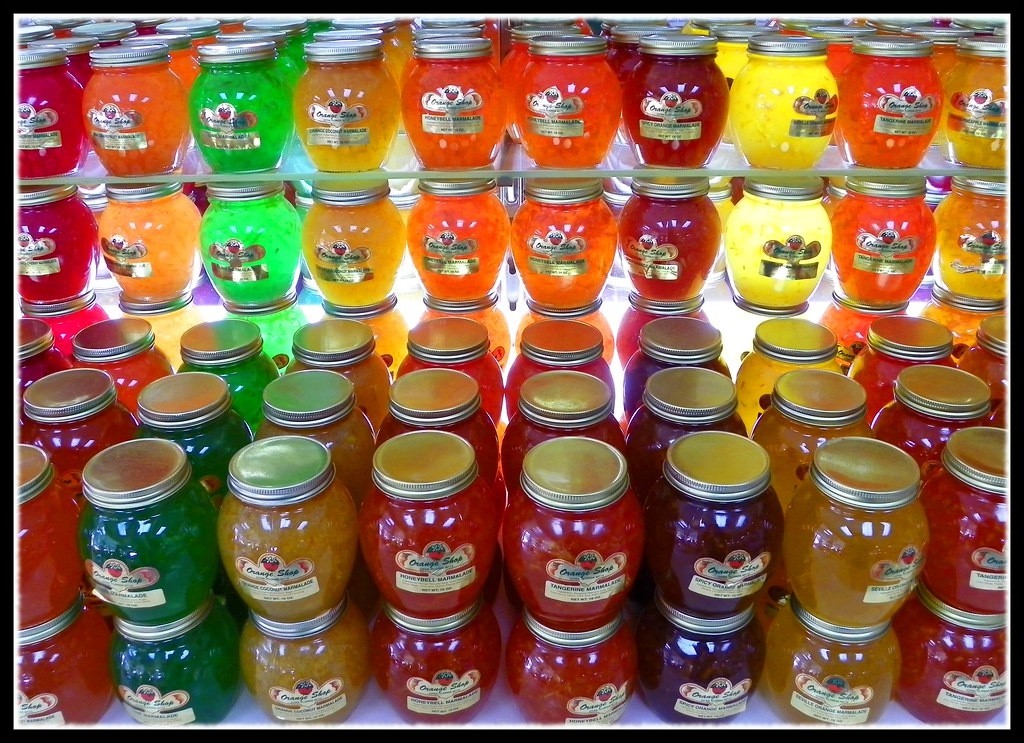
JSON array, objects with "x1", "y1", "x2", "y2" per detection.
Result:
[{"x1": 12, "y1": 10, "x2": 1012, "y2": 726}]
